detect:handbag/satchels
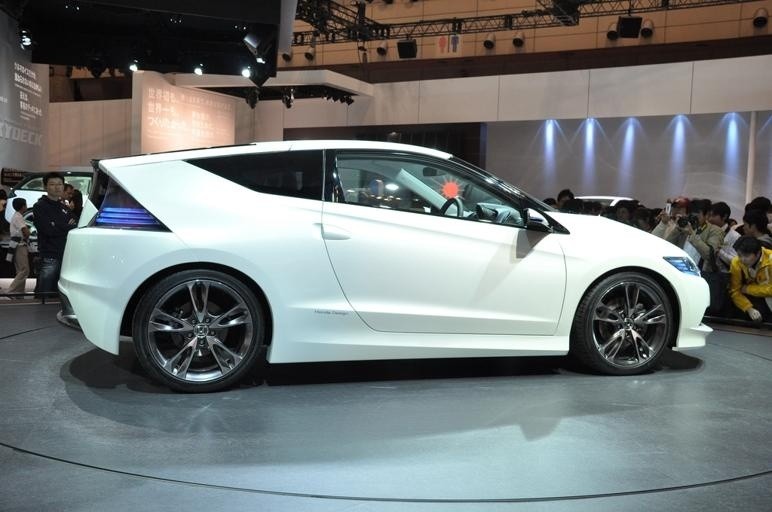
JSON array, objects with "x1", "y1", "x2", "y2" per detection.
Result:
[{"x1": 701, "y1": 244, "x2": 733, "y2": 313}]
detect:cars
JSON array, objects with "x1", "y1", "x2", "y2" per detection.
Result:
[{"x1": 4, "y1": 171, "x2": 94, "y2": 241}]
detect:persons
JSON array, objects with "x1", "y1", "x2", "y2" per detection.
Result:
[
  {"x1": 68, "y1": 188, "x2": 84, "y2": 220},
  {"x1": 31, "y1": 172, "x2": 78, "y2": 299},
  {"x1": 59, "y1": 182, "x2": 75, "y2": 207},
  {"x1": 543, "y1": 188, "x2": 772, "y2": 326},
  {"x1": 5, "y1": 198, "x2": 31, "y2": 302}
]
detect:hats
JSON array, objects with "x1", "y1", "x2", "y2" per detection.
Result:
[{"x1": 670, "y1": 196, "x2": 691, "y2": 208}]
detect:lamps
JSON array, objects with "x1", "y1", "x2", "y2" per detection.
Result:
[
  {"x1": 484, "y1": 33, "x2": 495, "y2": 49},
  {"x1": 377, "y1": 41, "x2": 388, "y2": 55},
  {"x1": 513, "y1": 31, "x2": 525, "y2": 46},
  {"x1": 244, "y1": 87, "x2": 355, "y2": 110},
  {"x1": 641, "y1": 19, "x2": 653, "y2": 37},
  {"x1": 305, "y1": 46, "x2": 314, "y2": 59},
  {"x1": 607, "y1": 23, "x2": 618, "y2": 40},
  {"x1": 752, "y1": 7, "x2": 768, "y2": 27},
  {"x1": 129, "y1": 32, "x2": 273, "y2": 87},
  {"x1": 282, "y1": 47, "x2": 293, "y2": 61}
]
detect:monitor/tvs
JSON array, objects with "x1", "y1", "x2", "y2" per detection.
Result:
[{"x1": 678, "y1": 216, "x2": 698, "y2": 230}]
